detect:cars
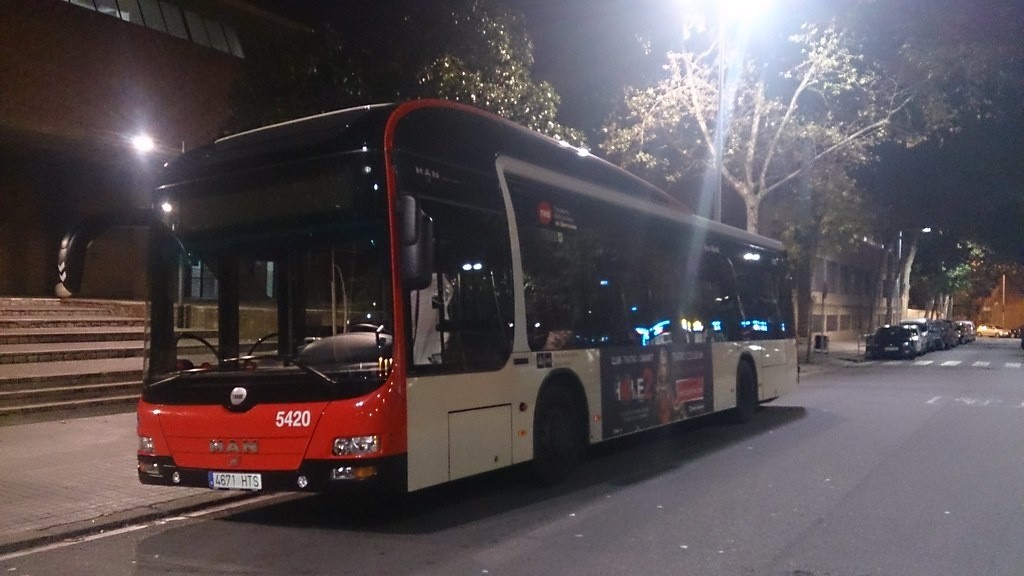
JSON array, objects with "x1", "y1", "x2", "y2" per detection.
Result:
[
  {"x1": 977, "y1": 324, "x2": 1010, "y2": 338},
  {"x1": 869, "y1": 325, "x2": 915, "y2": 359},
  {"x1": 899, "y1": 318, "x2": 976, "y2": 355},
  {"x1": 1010, "y1": 326, "x2": 1024, "y2": 339}
]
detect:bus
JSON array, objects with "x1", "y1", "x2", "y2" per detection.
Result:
[{"x1": 53, "y1": 99, "x2": 797, "y2": 509}]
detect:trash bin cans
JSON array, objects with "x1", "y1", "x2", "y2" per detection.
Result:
[
  {"x1": 177, "y1": 304, "x2": 191, "y2": 328},
  {"x1": 814, "y1": 335, "x2": 827, "y2": 350}
]
